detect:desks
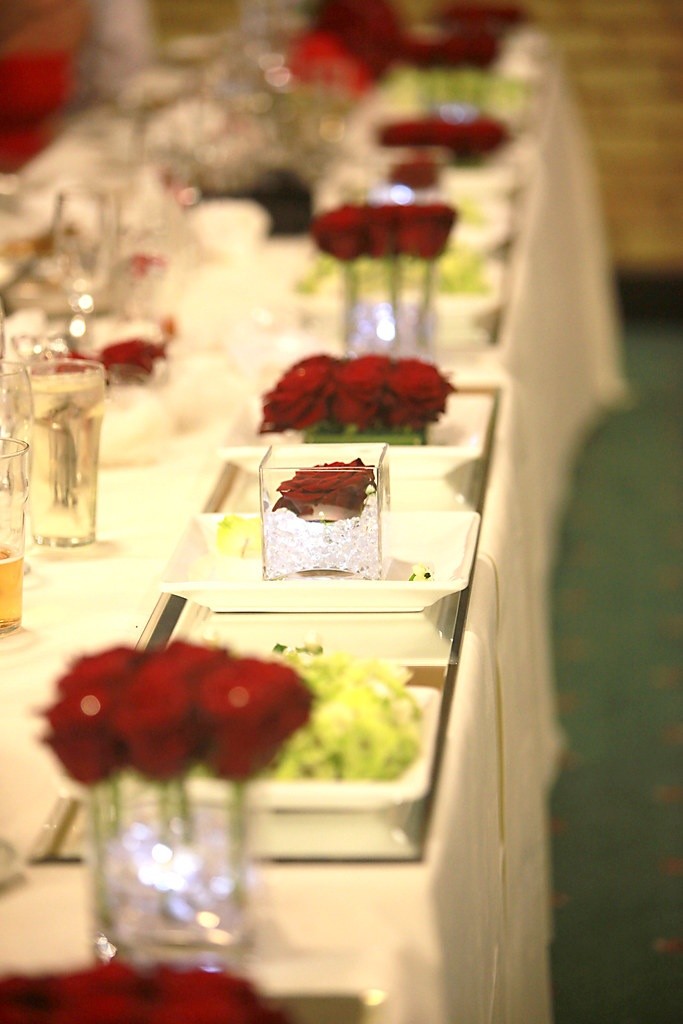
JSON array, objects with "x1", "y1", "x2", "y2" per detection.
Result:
[{"x1": 0, "y1": 22, "x2": 632, "y2": 1024}]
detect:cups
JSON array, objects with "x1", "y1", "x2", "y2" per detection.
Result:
[
  {"x1": 0, "y1": 359, "x2": 32, "y2": 542},
  {"x1": 0, "y1": 439, "x2": 29, "y2": 636},
  {"x1": 27, "y1": 362, "x2": 105, "y2": 548},
  {"x1": 56, "y1": 187, "x2": 121, "y2": 314}
]
